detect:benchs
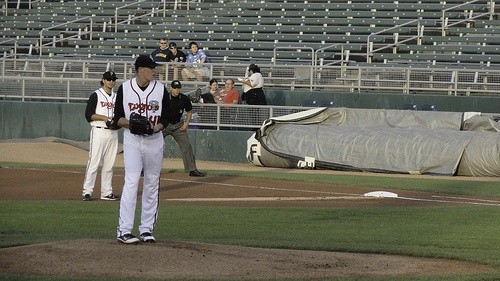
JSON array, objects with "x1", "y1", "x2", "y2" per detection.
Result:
[{"x1": 0, "y1": 0, "x2": 500, "y2": 98}]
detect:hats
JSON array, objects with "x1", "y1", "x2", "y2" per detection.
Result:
[
  {"x1": 134, "y1": 55, "x2": 162, "y2": 68},
  {"x1": 171, "y1": 80, "x2": 181, "y2": 88},
  {"x1": 248, "y1": 64, "x2": 258, "y2": 71},
  {"x1": 104, "y1": 71, "x2": 119, "y2": 79},
  {"x1": 169, "y1": 42, "x2": 177, "y2": 47}
]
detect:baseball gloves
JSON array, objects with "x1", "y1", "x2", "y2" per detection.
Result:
[
  {"x1": 105, "y1": 117, "x2": 122, "y2": 130},
  {"x1": 129, "y1": 112, "x2": 155, "y2": 136}
]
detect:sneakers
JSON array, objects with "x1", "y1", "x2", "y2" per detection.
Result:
[
  {"x1": 140, "y1": 232, "x2": 155, "y2": 242},
  {"x1": 83, "y1": 194, "x2": 90, "y2": 201},
  {"x1": 117, "y1": 234, "x2": 139, "y2": 243},
  {"x1": 101, "y1": 193, "x2": 119, "y2": 201}
]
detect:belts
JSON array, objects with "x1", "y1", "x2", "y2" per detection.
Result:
[{"x1": 169, "y1": 120, "x2": 180, "y2": 124}]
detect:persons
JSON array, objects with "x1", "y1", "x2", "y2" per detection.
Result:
[
  {"x1": 165, "y1": 42, "x2": 186, "y2": 71},
  {"x1": 114, "y1": 54, "x2": 171, "y2": 243},
  {"x1": 181, "y1": 42, "x2": 210, "y2": 87},
  {"x1": 215, "y1": 79, "x2": 239, "y2": 110},
  {"x1": 82, "y1": 71, "x2": 120, "y2": 200},
  {"x1": 203, "y1": 79, "x2": 218, "y2": 103},
  {"x1": 162, "y1": 80, "x2": 206, "y2": 177},
  {"x1": 240, "y1": 64, "x2": 268, "y2": 131},
  {"x1": 152, "y1": 38, "x2": 170, "y2": 80}
]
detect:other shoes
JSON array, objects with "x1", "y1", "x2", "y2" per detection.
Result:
[{"x1": 189, "y1": 170, "x2": 205, "y2": 177}]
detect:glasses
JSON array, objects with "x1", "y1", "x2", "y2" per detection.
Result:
[
  {"x1": 225, "y1": 83, "x2": 234, "y2": 85},
  {"x1": 173, "y1": 88, "x2": 181, "y2": 89},
  {"x1": 161, "y1": 42, "x2": 166, "y2": 44},
  {"x1": 170, "y1": 47, "x2": 176, "y2": 49},
  {"x1": 105, "y1": 78, "x2": 116, "y2": 82}
]
